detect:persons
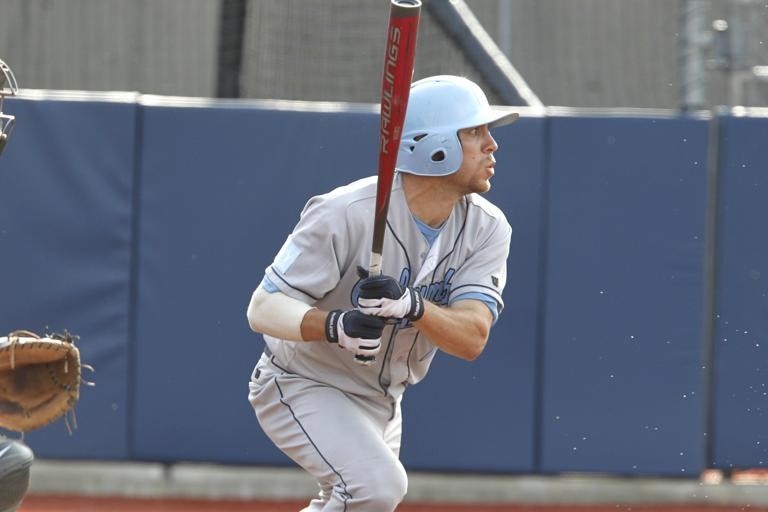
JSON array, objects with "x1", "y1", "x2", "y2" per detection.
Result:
[
  {"x1": 247, "y1": 70, "x2": 523, "y2": 511},
  {"x1": 1, "y1": 60, "x2": 82, "y2": 511}
]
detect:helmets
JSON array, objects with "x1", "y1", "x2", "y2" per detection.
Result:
[{"x1": 385, "y1": 72, "x2": 520, "y2": 178}]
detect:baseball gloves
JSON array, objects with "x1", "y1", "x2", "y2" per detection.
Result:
[{"x1": 1, "y1": 329, "x2": 93, "y2": 440}]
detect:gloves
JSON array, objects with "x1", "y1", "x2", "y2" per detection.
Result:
[
  {"x1": 356, "y1": 263, "x2": 424, "y2": 320},
  {"x1": 325, "y1": 307, "x2": 386, "y2": 353}
]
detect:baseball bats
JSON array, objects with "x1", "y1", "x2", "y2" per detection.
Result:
[{"x1": 355, "y1": 0, "x2": 423, "y2": 367}]
